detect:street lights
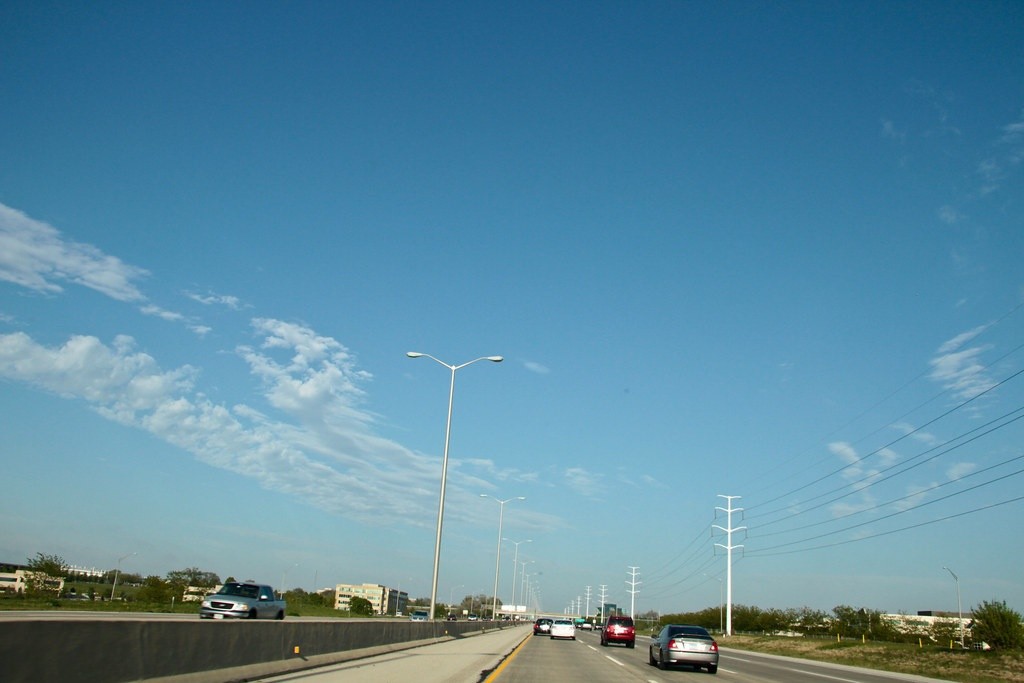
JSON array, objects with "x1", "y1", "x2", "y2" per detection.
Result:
[
  {"x1": 447, "y1": 585, "x2": 489, "y2": 617},
  {"x1": 939, "y1": 565, "x2": 968, "y2": 651},
  {"x1": 109, "y1": 552, "x2": 137, "y2": 601},
  {"x1": 402, "y1": 349, "x2": 504, "y2": 619},
  {"x1": 512, "y1": 559, "x2": 540, "y2": 613},
  {"x1": 500, "y1": 536, "x2": 532, "y2": 621}
]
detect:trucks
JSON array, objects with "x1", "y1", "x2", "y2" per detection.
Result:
[{"x1": 574, "y1": 619, "x2": 586, "y2": 629}]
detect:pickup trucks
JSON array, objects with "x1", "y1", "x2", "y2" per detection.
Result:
[{"x1": 200, "y1": 581, "x2": 288, "y2": 619}]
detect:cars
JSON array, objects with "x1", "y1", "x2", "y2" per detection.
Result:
[
  {"x1": 549, "y1": 619, "x2": 577, "y2": 641},
  {"x1": 467, "y1": 613, "x2": 479, "y2": 622},
  {"x1": 648, "y1": 624, "x2": 720, "y2": 674},
  {"x1": 410, "y1": 609, "x2": 430, "y2": 622},
  {"x1": 448, "y1": 613, "x2": 457, "y2": 622},
  {"x1": 581, "y1": 621, "x2": 592, "y2": 631},
  {"x1": 601, "y1": 615, "x2": 636, "y2": 648},
  {"x1": 595, "y1": 623, "x2": 605, "y2": 629},
  {"x1": 533, "y1": 617, "x2": 553, "y2": 635}
]
